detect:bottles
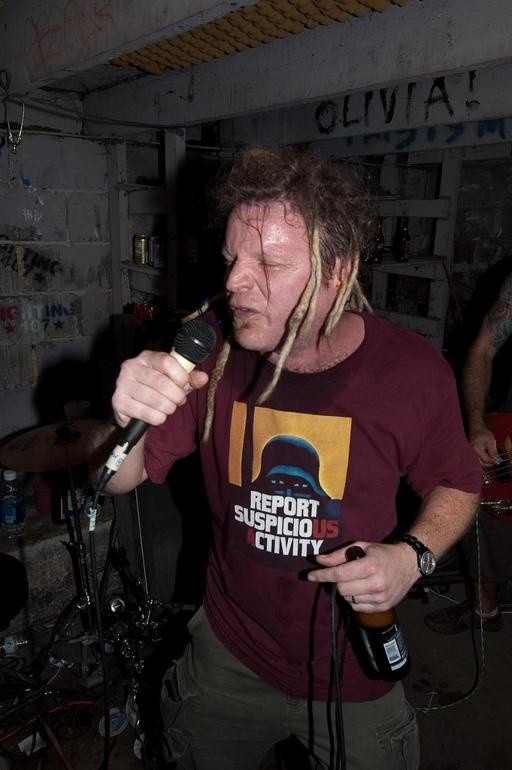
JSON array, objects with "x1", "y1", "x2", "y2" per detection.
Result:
[
  {"x1": 394, "y1": 216, "x2": 411, "y2": 263},
  {"x1": 371, "y1": 215, "x2": 385, "y2": 264},
  {"x1": 3, "y1": 619, "x2": 16, "y2": 658},
  {"x1": 343, "y1": 545, "x2": 411, "y2": 684},
  {"x1": 51, "y1": 467, "x2": 85, "y2": 527},
  {"x1": 1, "y1": 469, "x2": 28, "y2": 541}
]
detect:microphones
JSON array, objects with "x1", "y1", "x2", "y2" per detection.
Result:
[{"x1": 102, "y1": 318, "x2": 218, "y2": 482}]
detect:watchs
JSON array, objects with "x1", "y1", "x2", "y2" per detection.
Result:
[{"x1": 399, "y1": 535, "x2": 437, "y2": 576}]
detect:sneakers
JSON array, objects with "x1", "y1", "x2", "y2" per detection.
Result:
[{"x1": 421, "y1": 599, "x2": 505, "y2": 636}]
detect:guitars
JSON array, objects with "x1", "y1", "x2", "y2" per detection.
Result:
[{"x1": 476, "y1": 410, "x2": 511, "y2": 509}]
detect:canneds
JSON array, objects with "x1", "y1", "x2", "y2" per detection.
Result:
[{"x1": 132, "y1": 233, "x2": 163, "y2": 270}]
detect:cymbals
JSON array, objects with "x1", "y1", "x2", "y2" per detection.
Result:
[{"x1": 1, "y1": 420, "x2": 120, "y2": 473}]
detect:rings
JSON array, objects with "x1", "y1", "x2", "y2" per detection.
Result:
[{"x1": 351, "y1": 596, "x2": 355, "y2": 603}]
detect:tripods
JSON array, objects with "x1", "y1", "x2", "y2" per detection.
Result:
[{"x1": 11, "y1": 457, "x2": 96, "y2": 709}]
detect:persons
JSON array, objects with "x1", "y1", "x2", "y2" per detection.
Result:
[
  {"x1": 88, "y1": 145, "x2": 483, "y2": 770},
  {"x1": 424, "y1": 248, "x2": 512, "y2": 636}
]
